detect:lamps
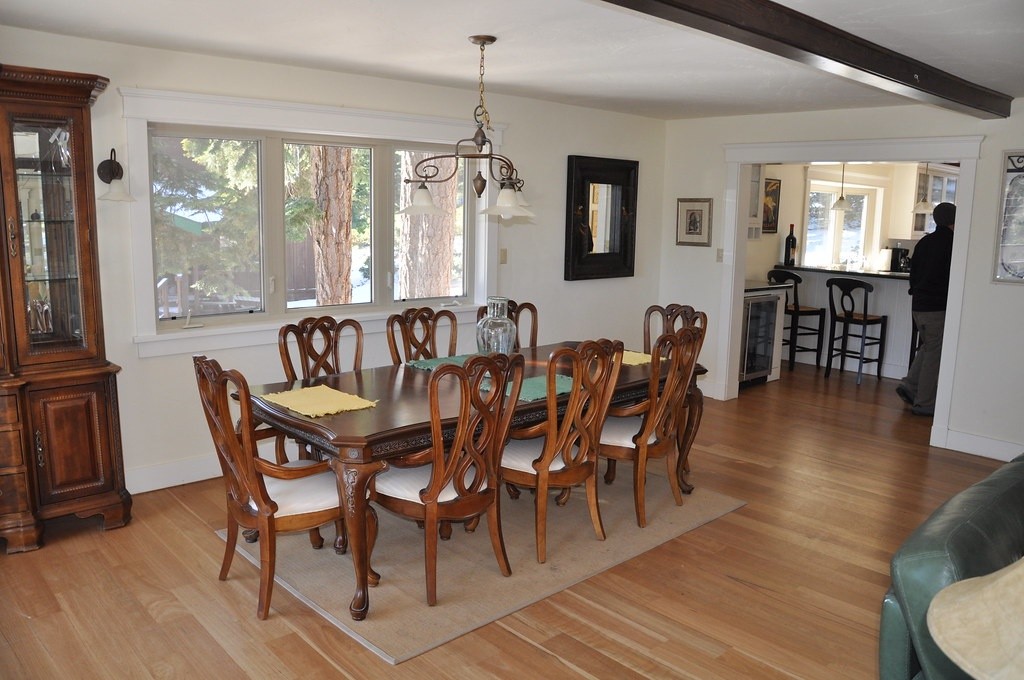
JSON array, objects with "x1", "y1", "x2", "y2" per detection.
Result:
[
  {"x1": 912, "y1": 164, "x2": 938, "y2": 213},
  {"x1": 830, "y1": 160, "x2": 852, "y2": 210},
  {"x1": 397, "y1": 35, "x2": 538, "y2": 218},
  {"x1": 95, "y1": 149, "x2": 136, "y2": 203}
]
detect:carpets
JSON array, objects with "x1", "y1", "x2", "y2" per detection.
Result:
[{"x1": 216, "y1": 443, "x2": 747, "y2": 665}]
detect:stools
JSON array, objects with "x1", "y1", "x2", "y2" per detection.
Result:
[
  {"x1": 767, "y1": 270, "x2": 825, "y2": 372},
  {"x1": 828, "y1": 277, "x2": 888, "y2": 387}
]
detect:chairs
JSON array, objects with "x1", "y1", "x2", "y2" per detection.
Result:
[
  {"x1": 465, "y1": 337, "x2": 627, "y2": 563},
  {"x1": 552, "y1": 327, "x2": 708, "y2": 527},
  {"x1": 273, "y1": 318, "x2": 373, "y2": 549},
  {"x1": 388, "y1": 307, "x2": 459, "y2": 364},
  {"x1": 478, "y1": 300, "x2": 539, "y2": 351},
  {"x1": 194, "y1": 356, "x2": 387, "y2": 619},
  {"x1": 644, "y1": 305, "x2": 713, "y2": 359},
  {"x1": 348, "y1": 354, "x2": 529, "y2": 606}
]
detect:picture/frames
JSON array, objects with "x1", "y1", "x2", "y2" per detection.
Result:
[
  {"x1": 761, "y1": 179, "x2": 781, "y2": 233},
  {"x1": 989, "y1": 150, "x2": 1024, "y2": 286},
  {"x1": 676, "y1": 196, "x2": 715, "y2": 246}
]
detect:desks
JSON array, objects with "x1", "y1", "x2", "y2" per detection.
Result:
[{"x1": 230, "y1": 344, "x2": 707, "y2": 620}]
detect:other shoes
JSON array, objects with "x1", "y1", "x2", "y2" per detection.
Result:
[
  {"x1": 897, "y1": 381, "x2": 914, "y2": 404},
  {"x1": 912, "y1": 403, "x2": 934, "y2": 416}
]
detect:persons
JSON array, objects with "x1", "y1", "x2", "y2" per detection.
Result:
[{"x1": 896, "y1": 202, "x2": 956, "y2": 417}]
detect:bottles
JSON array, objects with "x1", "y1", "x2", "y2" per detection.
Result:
[
  {"x1": 475, "y1": 296, "x2": 517, "y2": 378},
  {"x1": 784, "y1": 224, "x2": 797, "y2": 268}
]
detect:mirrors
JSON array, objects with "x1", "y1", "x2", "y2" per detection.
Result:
[{"x1": 563, "y1": 155, "x2": 641, "y2": 281}]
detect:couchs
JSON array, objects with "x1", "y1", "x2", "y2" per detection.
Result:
[{"x1": 875, "y1": 456, "x2": 1024, "y2": 680}]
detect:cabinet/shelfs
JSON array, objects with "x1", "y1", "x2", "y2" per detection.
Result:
[{"x1": 0, "y1": 64, "x2": 132, "y2": 551}]
